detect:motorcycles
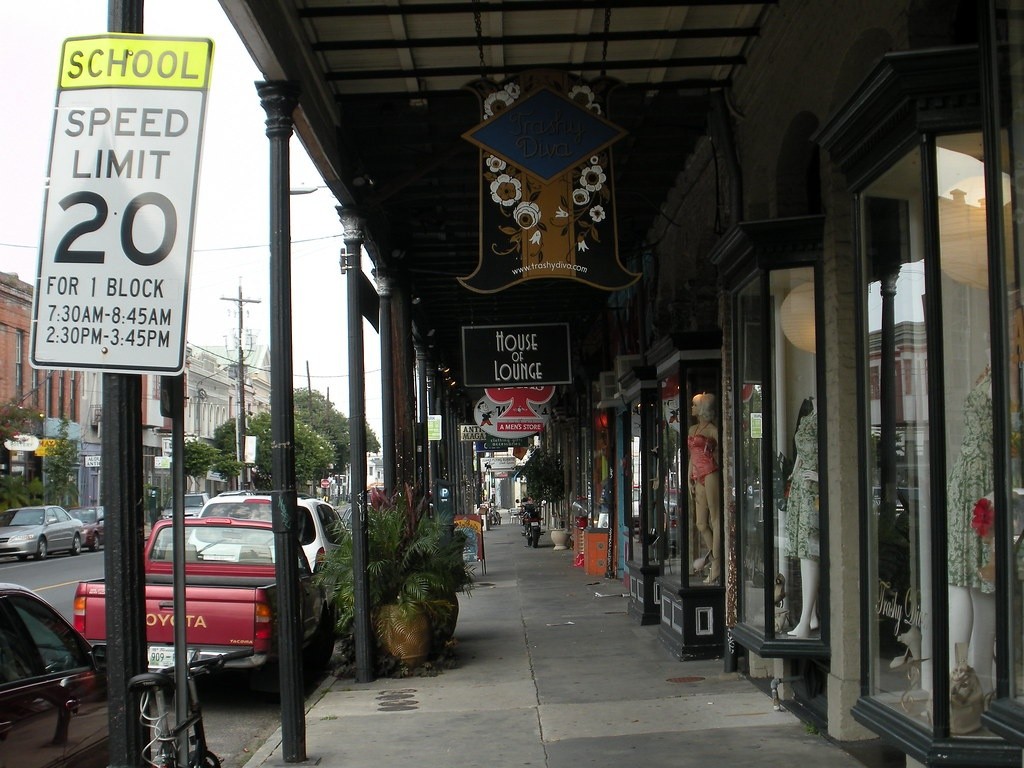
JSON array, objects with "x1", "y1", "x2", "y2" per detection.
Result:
[{"x1": 516, "y1": 498, "x2": 546, "y2": 548}]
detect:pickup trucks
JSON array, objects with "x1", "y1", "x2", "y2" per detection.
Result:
[{"x1": 71, "y1": 517, "x2": 337, "y2": 697}]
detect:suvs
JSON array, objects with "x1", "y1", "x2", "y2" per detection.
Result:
[
  {"x1": 160, "y1": 492, "x2": 211, "y2": 520},
  {"x1": 188, "y1": 487, "x2": 349, "y2": 575},
  {"x1": 215, "y1": 489, "x2": 253, "y2": 498}
]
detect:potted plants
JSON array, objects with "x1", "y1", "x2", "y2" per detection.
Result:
[
  {"x1": 512, "y1": 443, "x2": 569, "y2": 550},
  {"x1": 310, "y1": 481, "x2": 478, "y2": 677}
]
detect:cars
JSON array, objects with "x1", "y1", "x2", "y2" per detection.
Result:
[
  {"x1": 631, "y1": 484, "x2": 676, "y2": 545},
  {"x1": 872, "y1": 487, "x2": 909, "y2": 520},
  {"x1": 66, "y1": 505, "x2": 105, "y2": 552},
  {"x1": 334, "y1": 485, "x2": 386, "y2": 528},
  {"x1": 0, "y1": 505, "x2": 83, "y2": 562},
  {"x1": 0, "y1": 583, "x2": 111, "y2": 768}
]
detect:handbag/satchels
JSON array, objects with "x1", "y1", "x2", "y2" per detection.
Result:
[
  {"x1": 753, "y1": 603, "x2": 791, "y2": 632},
  {"x1": 926, "y1": 665, "x2": 985, "y2": 734}
]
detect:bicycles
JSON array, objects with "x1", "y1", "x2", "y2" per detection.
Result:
[
  {"x1": 490, "y1": 505, "x2": 502, "y2": 526},
  {"x1": 126, "y1": 645, "x2": 254, "y2": 768}
]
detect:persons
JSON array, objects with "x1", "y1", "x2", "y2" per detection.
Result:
[
  {"x1": 921, "y1": 346, "x2": 996, "y2": 718},
  {"x1": 786, "y1": 395, "x2": 821, "y2": 638},
  {"x1": 686, "y1": 392, "x2": 721, "y2": 583}
]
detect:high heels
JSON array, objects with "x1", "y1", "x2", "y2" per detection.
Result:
[
  {"x1": 774, "y1": 573, "x2": 786, "y2": 603},
  {"x1": 694, "y1": 549, "x2": 714, "y2": 578},
  {"x1": 702, "y1": 558, "x2": 721, "y2": 583}
]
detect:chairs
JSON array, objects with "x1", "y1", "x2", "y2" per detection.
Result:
[
  {"x1": 164, "y1": 543, "x2": 199, "y2": 561},
  {"x1": 239, "y1": 546, "x2": 273, "y2": 566}
]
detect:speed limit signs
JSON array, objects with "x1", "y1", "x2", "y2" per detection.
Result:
[{"x1": 30, "y1": 29, "x2": 212, "y2": 377}]
